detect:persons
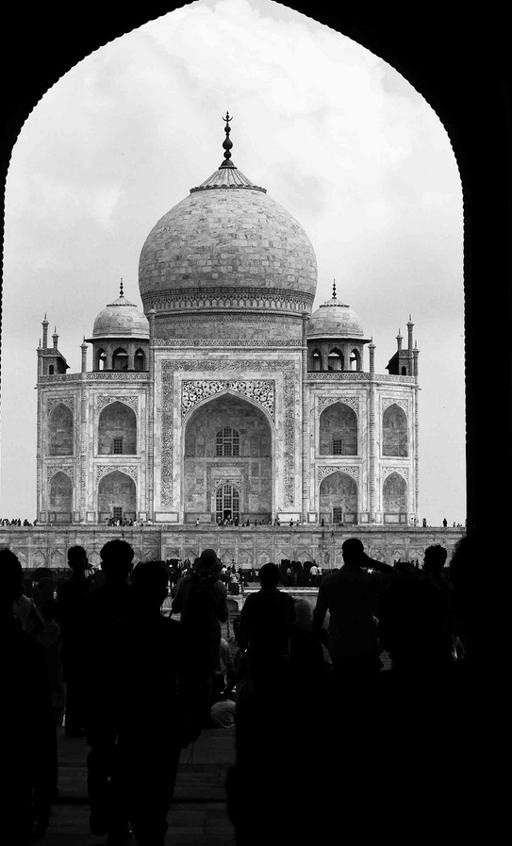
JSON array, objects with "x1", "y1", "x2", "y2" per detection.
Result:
[
  {"x1": 218, "y1": 518, "x2": 280, "y2": 526},
  {"x1": 196, "y1": 519, "x2": 200, "y2": 528},
  {"x1": 2, "y1": 518, "x2": 37, "y2": 527},
  {"x1": 289, "y1": 519, "x2": 300, "y2": 527},
  {"x1": 340, "y1": 519, "x2": 344, "y2": 526},
  {"x1": 108, "y1": 518, "x2": 152, "y2": 527},
  {"x1": 411, "y1": 518, "x2": 463, "y2": 528},
  {"x1": 2, "y1": 536, "x2": 511, "y2": 844}
]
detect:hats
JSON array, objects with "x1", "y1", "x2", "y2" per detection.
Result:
[{"x1": 194, "y1": 550, "x2": 223, "y2": 574}]
